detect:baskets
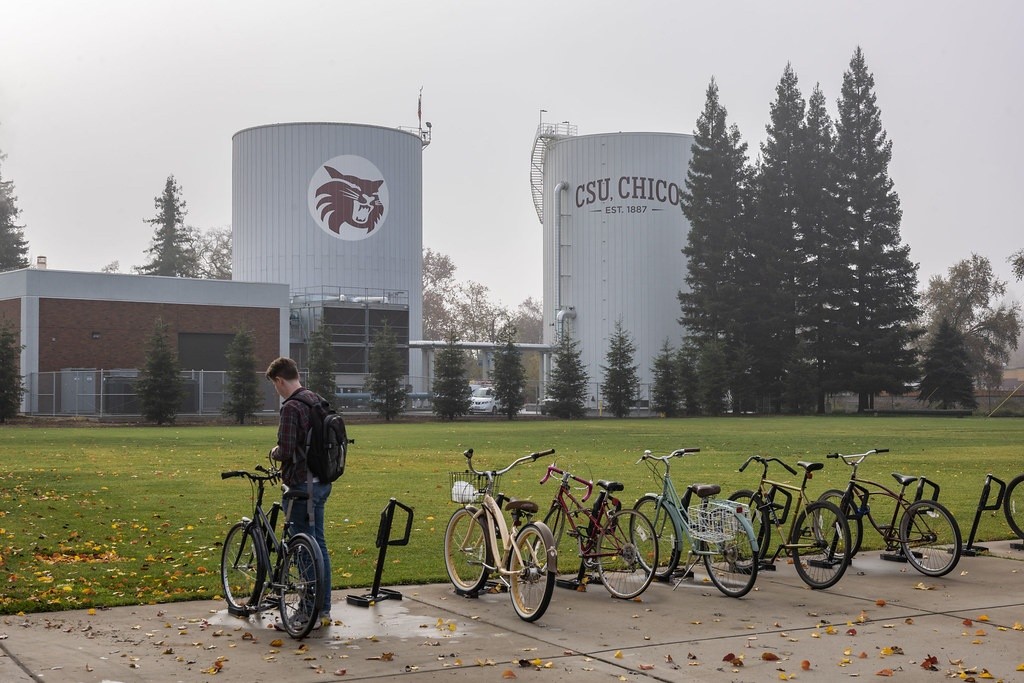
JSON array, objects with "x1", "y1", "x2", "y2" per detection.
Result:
[
  {"x1": 449, "y1": 472, "x2": 501, "y2": 504},
  {"x1": 687, "y1": 499, "x2": 754, "y2": 544}
]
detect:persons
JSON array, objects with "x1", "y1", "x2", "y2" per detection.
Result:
[{"x1": 265, "y1": 357, "x2": 332, "y2": 631}]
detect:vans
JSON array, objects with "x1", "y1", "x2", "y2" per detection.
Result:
[{"x1": 468, "y1": 387, "x2": 508, "y2": 415}]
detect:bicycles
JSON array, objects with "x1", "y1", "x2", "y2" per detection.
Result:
[
  {"x1": 723, "y1": 455, "x2": 853, "y2": 591},
  {"x1": 531, "y1": 458, "x2": 660, "y2": 600},
  {"x1": 1003, "y1": 473, "x2": 1024, "y2": 540},
  {"x1": 814, "y1": 448, "x2": 963, "y2": 576},
  {"x1": 220, "y1": 465, "x2": 327, "y2": 642},
  {"x1": 637, "y1": 446, "x2": 760, "y2": 598},
  {"x1": 442, "y1": 448, "x2": 559, "y2": 624}
]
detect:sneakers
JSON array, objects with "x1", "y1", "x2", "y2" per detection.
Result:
[
  {"x1": 318, "y1": 610, "x2": 332, "y2": 625},
  {"x1": 274, "y1": 611, "x2": 321, "y2": 630}
]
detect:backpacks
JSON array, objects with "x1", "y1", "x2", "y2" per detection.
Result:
[{"x1": 290, "y1": 392, "x2": 348, "y2": 485}]
